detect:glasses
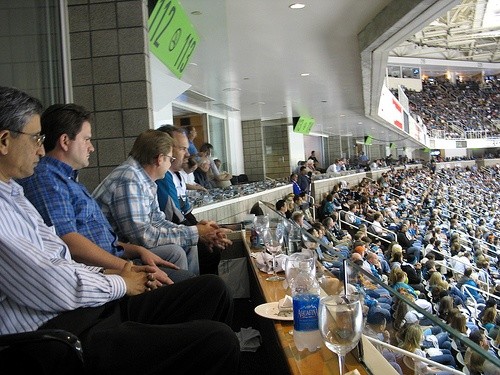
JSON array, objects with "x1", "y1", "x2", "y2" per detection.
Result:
[
  {"x1": 0, "y1": 128, "x2": 45, "y2": 147},
  {"x1": 164, "y1": 154, "x2": 176, "y2": 163}
]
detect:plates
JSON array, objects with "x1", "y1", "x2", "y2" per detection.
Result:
[{"x1": 254, "y1": 301, "x2": 295, "y2": 320}]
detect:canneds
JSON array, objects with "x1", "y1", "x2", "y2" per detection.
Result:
[{"x1": 250, "y1": 226, "x2": 263, "y2": 249}]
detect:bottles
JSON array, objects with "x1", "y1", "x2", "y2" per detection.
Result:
[{"x1": 293, "y1": 262, "x2": 321, "y2": 352}]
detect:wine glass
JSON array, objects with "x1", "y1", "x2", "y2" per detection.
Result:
[
  {"x1": 254, "y1": 215, "x2": 269, "y2": 249},
  {"x1": 286, "y1": 252, "x2": 316, "y2": 296},
  {"x1": 318, "y1": 295, "x2": 364, "y2": 375},
  {"x1": 264, "y1": 229, "x2": 285, "y2": 281}
]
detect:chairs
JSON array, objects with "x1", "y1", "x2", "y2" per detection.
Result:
[{"x1": 274, "y1": 73, "x2": 500, "y2": 375}]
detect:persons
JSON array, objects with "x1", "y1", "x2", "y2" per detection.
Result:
[
  {"x1": 91, "y1": 130, "x2": 233, "y2": 282},
  {"x1": 276, "y1": 76, "x2": 500, "y2": 375},
  {"x1": 12, "y1": 103, "x2": 194, "y2": 289},
  {"x1": 0, "y1": 85, "x2": 241, "y2": 375},
  {"x1": 156, "y1": 124, "x2": 221, "y2": 274},
  {"x1": 184, "y1": 120, "x2": 233, "y2": 195}
]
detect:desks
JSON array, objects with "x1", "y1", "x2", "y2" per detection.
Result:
[{"x1": 240, "y1": 224, "x2": 375, "y2": 374}]
